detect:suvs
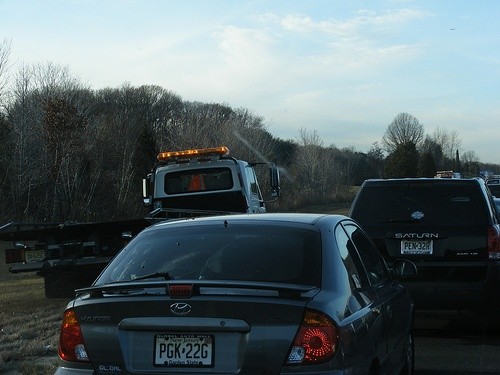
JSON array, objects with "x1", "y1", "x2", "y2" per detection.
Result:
[{"x1": 347, "y1": 178, "x2": 500, "y2": 335}]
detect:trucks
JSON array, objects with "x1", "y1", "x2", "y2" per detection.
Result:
[{"x1": 0, "y1": 147, "x2": 283, "y2": 298}]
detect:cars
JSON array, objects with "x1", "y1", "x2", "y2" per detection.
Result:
[{"x1": 54, "y1": 213, "x2": 420, "y2": 374}]
